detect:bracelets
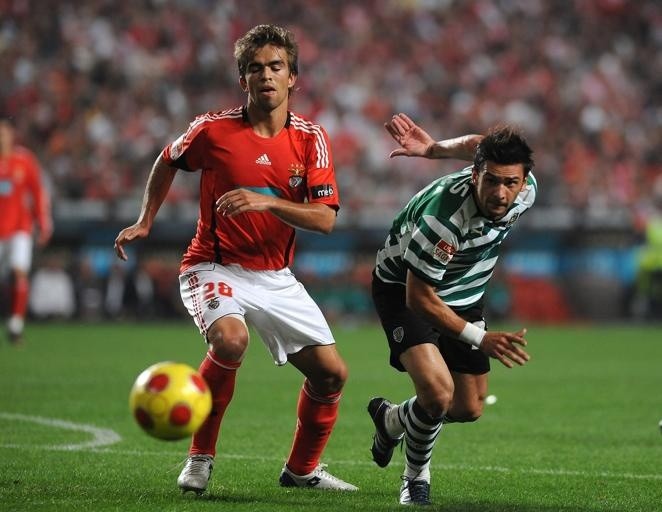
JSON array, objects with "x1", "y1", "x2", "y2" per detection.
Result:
[{"x1": 456, "y1": 322, "x2": 487, "y2": 348}]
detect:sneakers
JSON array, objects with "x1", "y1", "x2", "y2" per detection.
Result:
[
  {"x1": 8, "y1": 314, "x2": 24, "y2": 337},
  {"x1": 279, "y1": 462, "x2": 360, "y2": 491},
  {"x1": 177, "y1": 454, "x2": 216, "y2": 496},
  {"x1": 367, "y1": 397, "x2": 405, "y2": 468},
  {"x1": 399, "y1": 469, "x2": 432, "y2": 505}
]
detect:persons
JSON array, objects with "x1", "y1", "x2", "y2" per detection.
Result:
[
  {"x1": 3, "y1": 114, "x2": 56, "y2": 339},
  {"x1": 365, "y1": 111, "x2": 541, "y2": 505},
  {"x1": 112, "y1": 22, "x2": 359, "y2": 497},
  {"x1": 2, "y1": 0, "x2": 662, "y2": 321}
]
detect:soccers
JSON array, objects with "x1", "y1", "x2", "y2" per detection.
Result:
[{"x1": 126, "y1": 362, "x2": 213, "y2": 443}]
